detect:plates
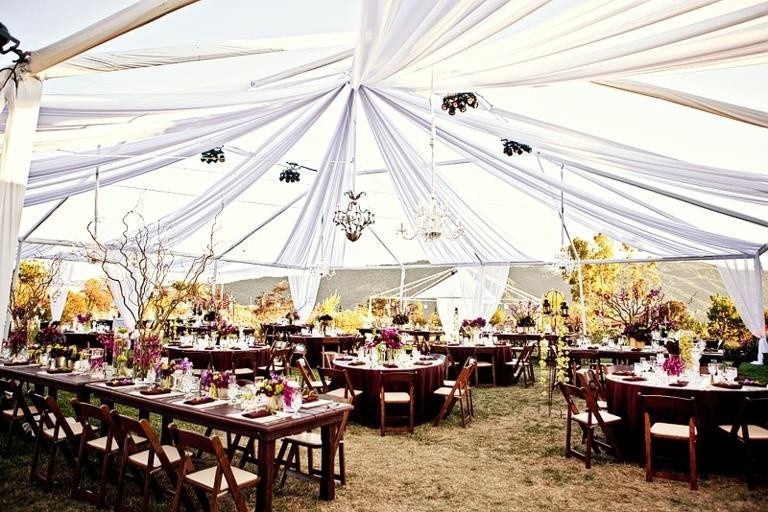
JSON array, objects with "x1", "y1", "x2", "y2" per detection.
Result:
[{"x1": 301, "y1": 399, "x2": 334, "y2": 409}]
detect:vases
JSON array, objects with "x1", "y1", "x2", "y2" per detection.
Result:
[
  {"x1": 115, "y1": 355, "x2": 127, "y2": 377},
  {"x1": 268, "y1": 394, "x2": 284, "y2": 412},
  {"x1": 382, "y1": 349, "x2": 397, "y2": 365},
  {"x1": 215, "y1": 384, "x2": 231, "y2": 402},
  {"x1": 44, "y1": 343, "x2": 55, "y2": 370},
  {"x1": 473, "y1": 331, "x2": 484, "y2": 347},
  {"x1": 136, "y1": 364, "x2": 151, "y2": 389},
  {"x1": 88, "y1": 348, "x2": 103, "y2": 376}
]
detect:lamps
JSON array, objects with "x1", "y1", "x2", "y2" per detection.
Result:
[
  {"x1": 397, "y1": 96, "x2": 467, "y2": 244},
  {"x1": 331, "y1": 93, "x2": 376, "y2": 243}
]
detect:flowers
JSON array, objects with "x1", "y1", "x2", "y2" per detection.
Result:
[
  {"x1": 113, "y1": 328, "x2": 134, "y2": 377},
  {"x1": 468, "y1": 317, "x2": 487, "y2": 333},
  {"x1": 255, "y1": 376, "x2": 293, "y2": 400},
  {"x1": 35, "y1": 327, "x2": 64, "y2": 348},
  {"x1": 664, "y1": 339, "x2": 692, "y2": 376},
  {"x1": 198, "y1": 365, "x2": 232, "y2": 394},
  {"x1": 130, "y1": 330, "x2": 162, "y2": 371},
  {"x1": 371, "y1": 330, "x2": 401, "y2": 353}
]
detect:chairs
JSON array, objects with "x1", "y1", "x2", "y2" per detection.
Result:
[
  {"x1": 432, "y1": 355, "x2": 477, "y2": 429},
  {"x1": 225, "y1": 349, "x2": 257, "y2": 382},
  {"x1": 278, "y1": 393, "x2": 354, "y2": 500},
  {"x1": 0, "y1": 377, "x2": 49, "y2": 455},
  {"x1": 68, "y1": 397, "x2": 150, "y2": 505},
  {"x1": 107, "y1": 408, "x2": 195, "y2": 511},
  {"x1": 180, "y1": 349, "x2": 214, "y2": 371},
  {"x1": 432, "y1": 344, "x2": 459, "y2": 377},
  {"x1": 315, "y1": 366, "x2": 364, "y2": 431},
  {"x1": 505, "y1": 344, "x2": 531, "y2": 387},
  {"x1": 320, "y1": 340, "x2": 345, "y2": 369},
  {"x1": 196, "y1": 423, "x2": 302, "y2": 475},
  {"x1": 256, "y1": 347, "x2": 292, "y2": 378},
  {"x1": 569, "y1": 348, "x2": 603, "y2": 387},
  {"x1": 168, "y1": 422, "x2": 262, "y2": 511},
  {"x1": 280, "y1": 340, "x2": 305, "y2": 363},
  {"x1": 378, "y1": 370, "x2": 419, "y2": 437},
  {"x1": 718, "y1": 393, "x2": 768, "y2": 488},
  {"x1": 637, "y1": 390, "x2": 698, "y2": 488},
  {"x1": 557, "y1": 379, "x2": 623, "y2": 471},
  {"x1": 162, "y1": 348, "x2": 171, "y2": 361},
  {"x1": 27, "y1": 387, "x2": 100, "y2": 490},
  {"x1": 296, "y1": 354, "x2": 332, "y2": 396},
  {"x1": 512, "y1": 341, "x2": 536, "y2": 385},
  {"x1": 577, "y1": 366, "x2": 610, "y2": 412},
  {"x1": 471, "y1": 345, "x2": 497, "y2": 386}
]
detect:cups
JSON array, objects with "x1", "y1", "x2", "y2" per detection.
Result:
[
  {"x1": 640, "y1": 358, "x2": 646, "y2": 366},
  {"x1": 618, "y1": 338, "x2": 624, "y2": 351},
  {"x1": 717, "y1": 362, "x2": 725, "y2": 383},
  {"x1": 707, "y1": 363, "x2": 717, "y2": 383},
  {"x1": 711, "y1": 359, "x2": 717, "y2": 368},
  {"x1": 726, "y1": 367, "x2": 738, "y2": 384},
  {"x1": 291, "y1": 392, "x2": 302, "y2": 418},
  {"x1": 650, "y1": 356, "x2": 655, "y2": 365},
  {"x1": 643, "y1": 361, "x2": 650, "y2": 375},
  {"x1": 635, "y1": 363, "x2": 642, "y2": 376},
  {"x1": 254, "y1": 377, "x2": 264, "y2": 391},
  {"x1": 725, "y1": 362, "x2": 732, "y2": 373}
]
